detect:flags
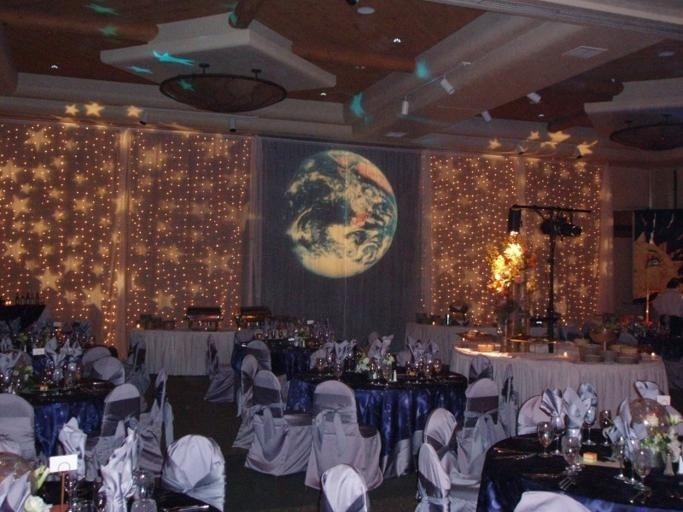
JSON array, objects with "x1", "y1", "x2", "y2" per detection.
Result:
[{"x1": 502, "y1": 266, "x2": 531, "y2": 353}]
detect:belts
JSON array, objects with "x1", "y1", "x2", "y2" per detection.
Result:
[
  {"x1": 61, "y1": 473, "x2": 79, "y2": 509},
  {"x1": 536, "y1": 399, "x2": 683, "y2": 505},
  {"x1": 256, "y1": 312, "x2": 452, "y2": 386},
  {"x1": 0, "y1": 361, "x2": 83, "y2": 395}
]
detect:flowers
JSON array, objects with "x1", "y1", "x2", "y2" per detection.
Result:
[{"x1": 483, "y1": 240, "x2": 540, "y2": 331}]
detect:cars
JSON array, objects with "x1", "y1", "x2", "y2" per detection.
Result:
[
  {"x1": 69, "y1": 492, "x2": 106, "y2": 512},
  {"x1": 131, "y1": 472, "x2": 157, "y2": 512}
]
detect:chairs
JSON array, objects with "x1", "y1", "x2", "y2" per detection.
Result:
[{"x1": 0, "y1": 315, "x2": 682, "y2": 511}]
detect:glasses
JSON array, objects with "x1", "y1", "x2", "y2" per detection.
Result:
[
  {"x1": 134, "y1": 103, "x2": 238, "y2": 135},
  {"x1": 606, "y1": 114, "x2": 683, "y2": 155},
  {"x1": 502, "y1": 202, "x2": 594, "y2": 242},
  {"x1": 479, "y1": 92, "x2": 542, "y2": 124},
  {"x1": 154, "y1": 58, "x2": 290, "y2": 116},
  {"x1": 397, "y1": 68, "x2": 457, "y2": 118}
]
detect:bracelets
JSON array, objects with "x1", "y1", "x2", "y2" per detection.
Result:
[{"x1": 578, "y1": 343, "x2": 640, "y2": 364}]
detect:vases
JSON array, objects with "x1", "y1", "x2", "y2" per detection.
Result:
[
  {"x1": 502, "y1": 266, "x2": 531, "y2": 353},
  {"x1": 483, "y1": 240, "x2": 540, "y2": 331}
]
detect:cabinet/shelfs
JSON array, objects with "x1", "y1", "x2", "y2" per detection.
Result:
[
  {"x1": 599, "y1": 328, "x2": 610, "y2": 354},
  {"x1": 14, "y1": 291, "x2": 39, "y2": 305},
  {"x1": 9, "y1": 329, "x2": 95, "y2": 354}
]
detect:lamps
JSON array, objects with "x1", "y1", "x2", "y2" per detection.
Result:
[
  {"x1": 154, "y1": 58, "x2": 290, "y2": 116},
  {"x1": 502, "y1": 202, "x2": 594, "y2": 242},
  {"x1": 134, "y1": 103, "x2": 238, "y2": 135},
  {"x1": 479, "y1": 92, "x2": 542, "y2": 124},
  {"x1": 397, "y1": 68, "x2": 457, "y2": 118},
  {"x1": 606, "y1": 114, "x2": 683, "y2": 155}
]
